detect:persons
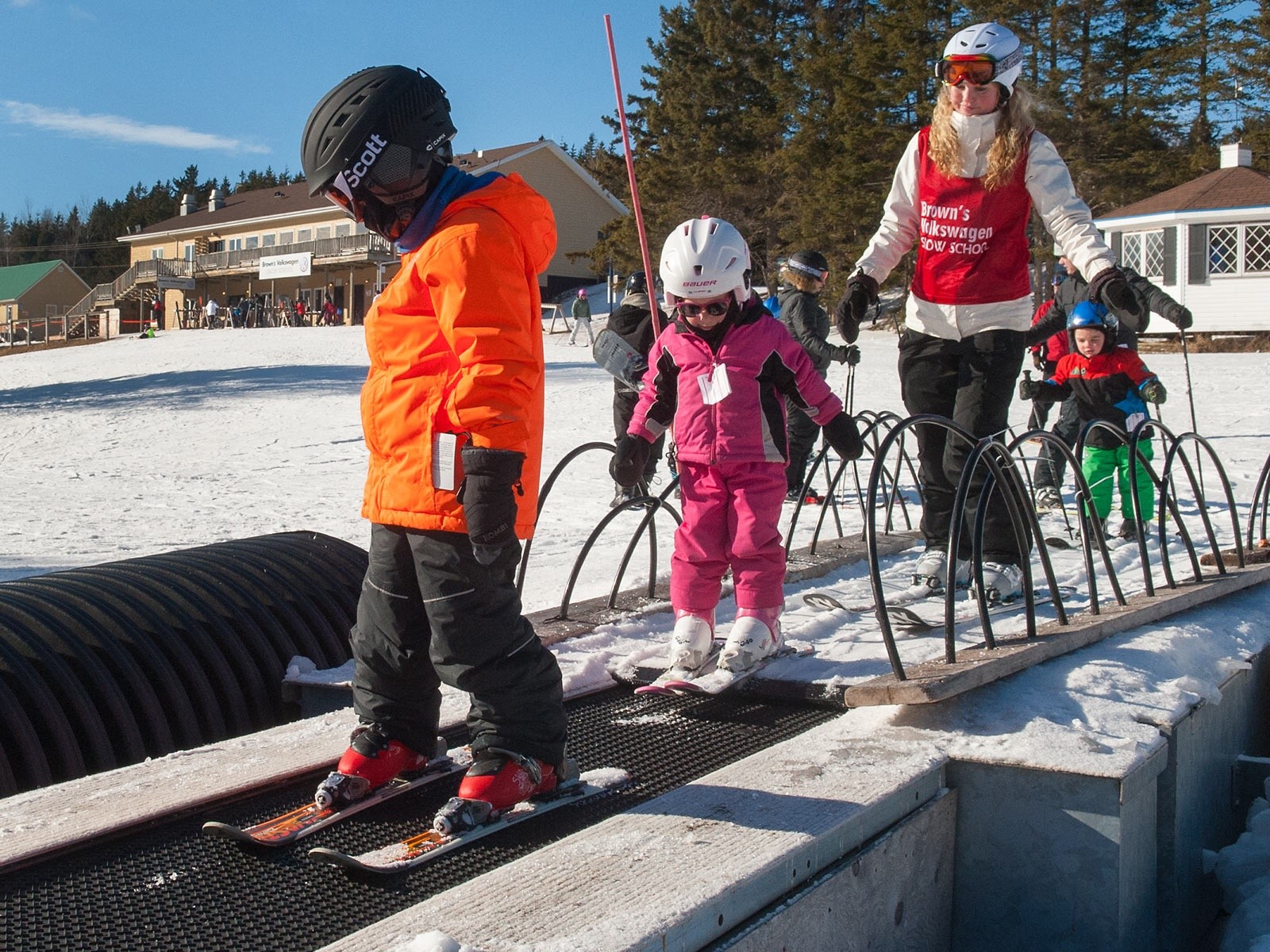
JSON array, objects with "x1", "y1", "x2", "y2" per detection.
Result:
[
  {"x1": 180, "y1": 284, "x2": 358, "y2": 335},
  {"x1": 290, "y1": 61, "x2": 568, "y2": 837},
  {"x1": 837, "y1": 15, "x2": 1146, "y2": 603},
  {"x1": 604, "y1": 212, "x2": 869, "y2": 679},
  {"x1": 1016, "y1": 240, "x2": 1192, "y2": 513},
  {"x1": 757, "y1": 249, "x2": 864, "y2": 504},
  {"x1": 568, "y1": 287, "x2": 596, "y2": 350},
  {"x1": 1024, "y1": 269, "x2": 1069, "y2": 442},
  {"x1": 134, "y1": 323, "x2": 155, "y2": 340},
  {"x1": 605, "y1": 272, "x2": 669, "y2": 513},
  {"x1": 152, "y1": 300, "x2": 166, "y2": 331},
  {"x1": 1019, "y1": 298, "x2": 1167, "y2": 540}
]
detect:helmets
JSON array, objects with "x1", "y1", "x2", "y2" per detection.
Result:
[
  {"x1": 659, "y1": 215, "x2": 752, "y2": 309},
  {"x1": 578, "y1": 289, "x2": 587, "y2": 301},
  {"x1": 300, "y1": 65, "x2": 457, "y2": 198},
  {"x1": 787, "y1": 250, "x2": 828, "y2": 281},
  {"x1": 626, "y1": 271, "x2": 657, "y2": 295},
  {"x1": 943, "y1": 22, "x2": 1024, "y2": 109},
  {"x1": 1068, "y1": 299, "x2": 1118, "y2": 356}
]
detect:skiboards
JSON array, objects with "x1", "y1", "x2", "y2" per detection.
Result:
[
  {"x1": 630, "y1": 641, "x2": 811, "y2": 703},
  {"x1": 804, "y1": 562, "x2": 1076, "y2": 630},
  {"x1": 1046, "y1": 524, "x2": 1162, "y2": 555},
  {"x1": 777, "y1": 487, "x2": 910, "y2": 509},
  {"x1": 183, "y1": 737, "x2": 632, "y2": 872}
]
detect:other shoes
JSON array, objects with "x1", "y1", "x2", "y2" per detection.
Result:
[
  {"x1": 1034, "y1": 485, "x2": 1149, "y2": 543},
  {"x1": 433, "y1": 750, "x2": 558, "y2": 839},
  {"x1": 909, "y1": 549, "x2": 973, "y2": 595},
  {"x1": 672, "y1": 609, "x2": 714, "y2": 671},
  {"x1": 609, "y1": 479, "x2": 654, "y2": 510},
  {"x1": 315, "y1": 728, "x2": 427, "y2": 813},
  {"x1": 713, "y1": 605, "x2": 787, "y2": 673},
  {"x1": 965, "y1": 560, "x2": 1027, "y2": 608},
  {"x1": 784, "y1": 482, "x2": 821, "y2": 504}
]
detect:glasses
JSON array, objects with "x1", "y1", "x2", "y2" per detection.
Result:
[
  {"x1": 934, "y1": 54, "x2": 997, "y2": 86},
  {"x1": 819, "y1": 271, "x2": 829, "y2": 283},
  {"x1": 674, "y1": 294, "x2": 733, "y2": 316},
  {"x1": 1050, "y1": 274, "x2": 1068, "y2": 286},
  {"x1": 323, "y1": 183, "x2": 366, "y2": 224}
]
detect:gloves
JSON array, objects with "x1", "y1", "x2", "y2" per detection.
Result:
[
  {"x1": 847, "y1": 344, "x2": 861, "y2": 367},
  {"x1": 823, "y1": 411, "x2": 862, "y2": 461},
  {"x1": 612, "y1": 434, "x2": 648, "y2": 481},
  {"x1": 1034, "y1": 355, "x2": 1045, "y2": 372},
  {"x1": 1101, "y1": 278, "x2": 1149, "y2": 333},
  {"x1": 1020, "y1": 380, "x2": 1040, "y2": 400},
  {"x1": 835, "y1": 281, "x2": 871, "y2": 344},
  {"x1": 458, "y1": 447, "x2": 527, "y2": 566},
  {"x1": 1142, "y1": 379, "x2": 1167, "y2": 405},
  {"x1": 1172, "y1": 306, "x2": 1192, "y2": 329}
]
